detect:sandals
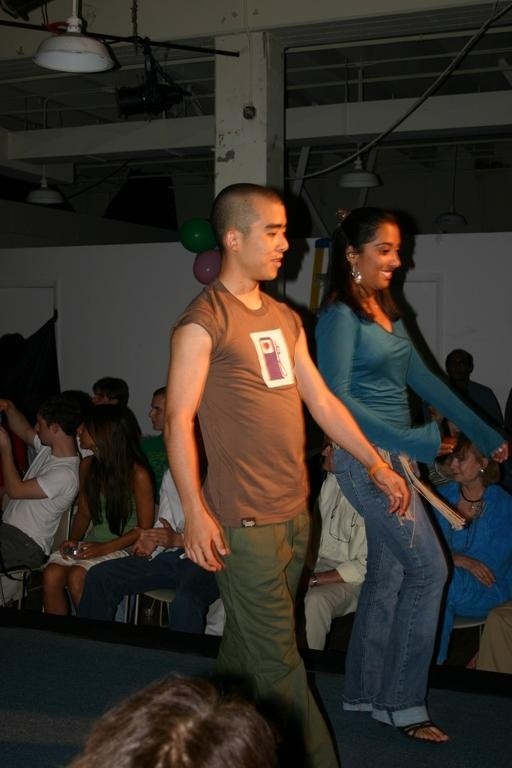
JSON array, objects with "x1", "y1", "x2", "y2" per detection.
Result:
[{"x1": 381, "y1": 718, "x2": 449, "y2": 743}]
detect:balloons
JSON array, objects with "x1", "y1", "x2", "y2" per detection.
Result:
[{"x1": 177, "y1": 217, "x2": 223, "y2": 285}]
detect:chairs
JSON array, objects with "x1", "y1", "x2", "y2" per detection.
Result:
[{"x1": 2, "y1": 498, "x2": 80, "y2": 611}]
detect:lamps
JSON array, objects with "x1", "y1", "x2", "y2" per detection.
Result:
[
  {"x1": 25, "y1": 93, "x2": 67, "y2": 206},
  {"x1": 338, "y1": 60, "x2": 387, "y2": 189},
  {"x1": 431, "y1": 139, "x2": 471, "y2": 226},
  {"x1": 112, "y1": 40, "x2": 189, "y2": 118},
  {"x1": 32, "y1": 0, "x2": 118, "y2": 77}
]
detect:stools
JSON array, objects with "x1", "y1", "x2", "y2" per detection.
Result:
[
  {"x1": 451, "y1": 614, "x2": 486, "y2": 639},
  {"x1": 132, "y1": 588, "x2": 178, "y2": 628}
]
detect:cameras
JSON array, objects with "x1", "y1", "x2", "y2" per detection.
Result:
[{"x1": 63, "y1": 546, "x2": 83, "y2": 556}]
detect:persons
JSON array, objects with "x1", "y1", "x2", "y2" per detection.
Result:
[
  {"x1": 162, "y1": 182, "x2": 410, "y2": 768},
  {"x1": 315, "y1": 204, "x2": 509, "y2": 745},
  {"x1": 63, "y1": 674, "x2": 281, "y2": 768}
]
